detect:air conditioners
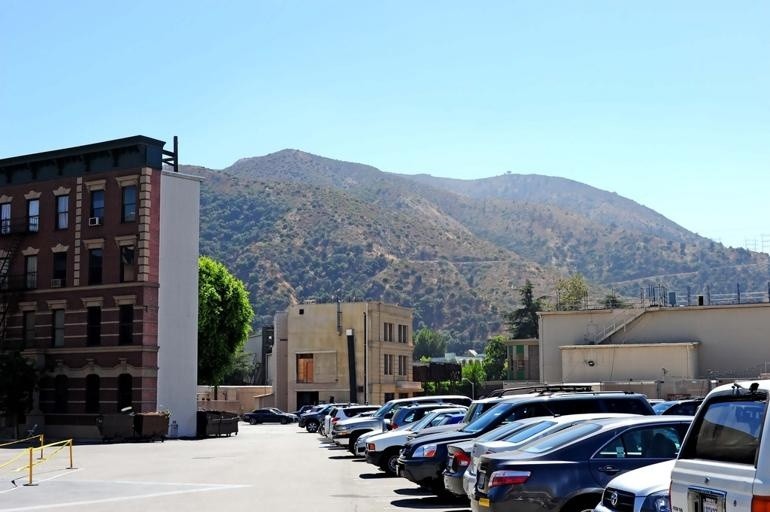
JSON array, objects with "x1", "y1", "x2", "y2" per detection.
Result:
[{"x1": 89, "y1": 217, "x2": 99, "y2": 226}]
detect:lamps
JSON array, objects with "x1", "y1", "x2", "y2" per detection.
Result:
[{"x1": 51, "y1": 279, "x2": 61, "y2": 288}]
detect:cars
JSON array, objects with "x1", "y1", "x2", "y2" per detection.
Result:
[{"x1": 241, "y1": 407, "x2": 297, "y2": 426}]
